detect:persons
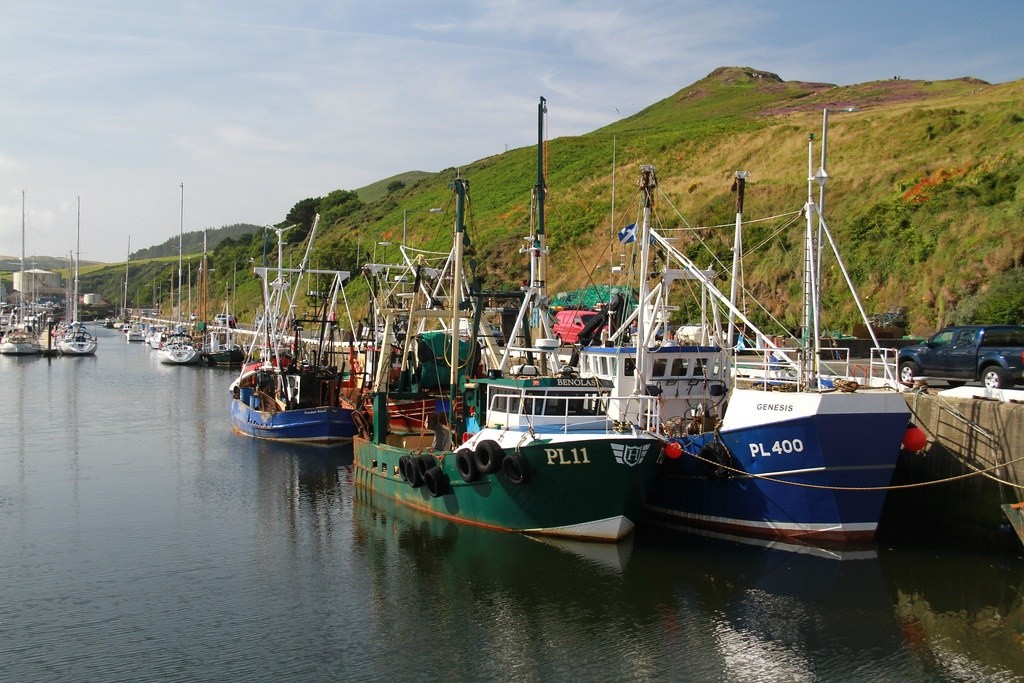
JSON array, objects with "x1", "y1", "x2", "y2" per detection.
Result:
[
  {"x1": 354, "y1": 320, "x2": 363, "y2": 339},
  {"x1": 630, "y1": 319, "x2": 638, "y2": 333},
  {"x1": 229, "y1": 313, "x2": 237, "y2": 327}
]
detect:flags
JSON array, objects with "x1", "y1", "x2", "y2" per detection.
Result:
[{"x1": 618, "y1": 224, "x2": 637, "y2": 244}]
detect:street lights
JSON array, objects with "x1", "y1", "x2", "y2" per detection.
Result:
[
  {"x1": 402, "y1": 208, "x2": 442, "y2": 310},
  {"x1": 159, "y1": 279, "x2": 171, "y2": 317},
  {"x1": 813, "y1": 105, "x2": 861, "y2": 356},
  {"x1": 373, "y1": 240, "x2": 391, "y2": 264}
]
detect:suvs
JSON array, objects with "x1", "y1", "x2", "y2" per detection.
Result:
[{"x1": 447, "y1": 317, "x2": 503, "y2": 348}]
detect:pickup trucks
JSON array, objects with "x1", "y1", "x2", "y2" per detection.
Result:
[{"x1": 894, "y1": 325, "x2": 1024, "y2": 388}]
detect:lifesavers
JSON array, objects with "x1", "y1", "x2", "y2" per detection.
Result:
[
  {"x1": 474, "y1": 439, "x2": 503, "y2": 474},
  {"x1": 698, "y1": 444, "x2": 732, "y2": 482},
  {"x1": 398, "y1": 455, "x2": 410, "y2": 483},
  {"x1": 416, "y1": 455, "x2": 436, "y2": 480},
  {"x1": 456, "y1": 448, "x2": 479, "y2": 483},
  {"x1": 423, "y1": 467, "x2": 446, "y2": 498},
  {"x1": 405, "y1": 457, "x2": 426, "y2": 488},
  {"x1": 502, "y1": 453, "x2": 531, "y2": 485}
]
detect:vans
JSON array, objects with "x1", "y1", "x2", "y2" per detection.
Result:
[{"x1": 553, "y1": 310, "x2": 609, "y2": 347}]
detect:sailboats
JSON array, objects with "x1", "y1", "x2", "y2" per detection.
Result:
[
  {"x1": 1, "y1": 189, "x2": 98, "y2": 354},
  {"x1": 102, "y1": 181, "x2": 242, "y2": 368}
]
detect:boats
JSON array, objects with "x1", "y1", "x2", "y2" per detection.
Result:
[{"x1": 228, "y1": 95, "x2": 915, "y2": 546}]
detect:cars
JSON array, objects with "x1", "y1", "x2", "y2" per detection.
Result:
[
  {"x1": 190, "y1": 313, "x2": 199, "y2": 321},
  {"x1": 142, "y1": 309, "x2": 157, "y2": 316},
  {"x1": 214, "y1": 314, "x2": 226, "y2": 323}
]
